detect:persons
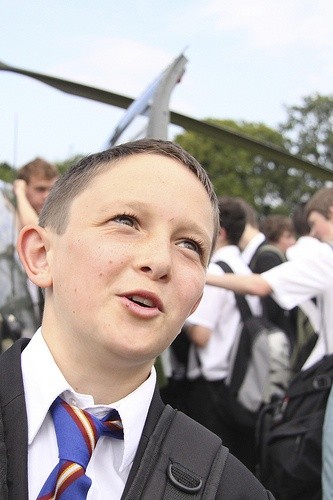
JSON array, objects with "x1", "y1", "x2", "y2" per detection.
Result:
[
  {"x1": 155, "y1": 188, "x2": 333, "y2": 500},
  {"x1": 0, "y1": 138, "x2": 275, "y2": 500},
  {"x1": 0, "y1": 158, "x2": 57, "y2": 338}
]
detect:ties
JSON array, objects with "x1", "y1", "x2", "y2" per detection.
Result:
[
  {"x1": 38, "y1": 286, "x2": 45, "y2": 324},
  {"x1": 36, "y1": 395, "x2": 124, "y2": 500}
]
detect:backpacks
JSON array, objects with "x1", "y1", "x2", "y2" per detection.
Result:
[
  {"x1": 236, "y1": 352, "x2": 333, "y2": 500},
  {"x1": 249, "y1": 245, "x2": 319, "y2": 371},
  {"x1": 194, "y1": 260, "x2": 292, "y2": 425}
]
